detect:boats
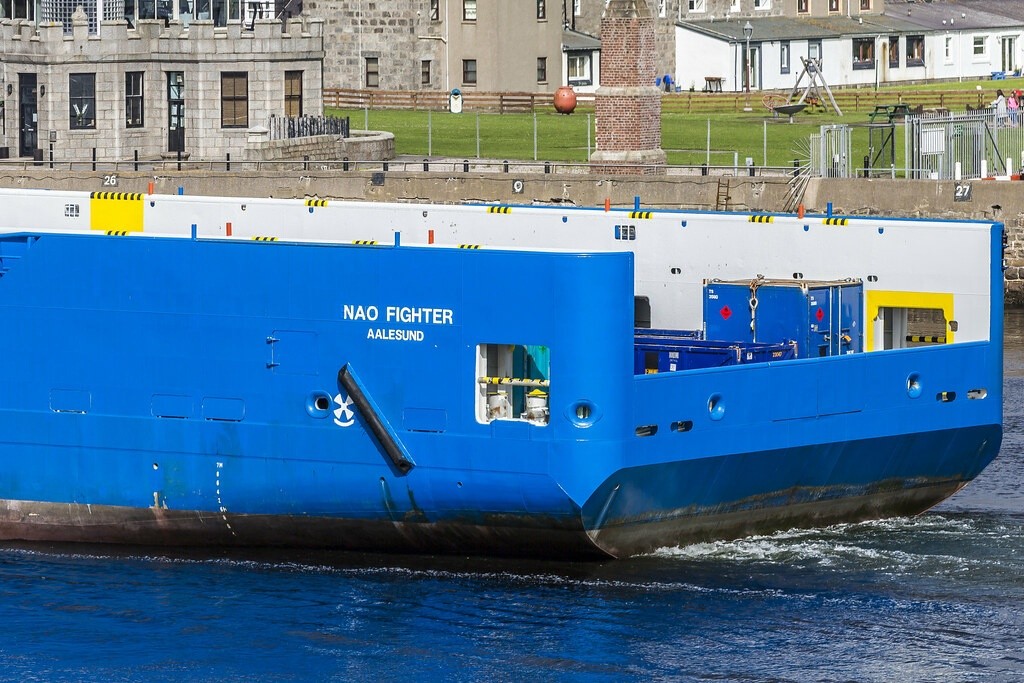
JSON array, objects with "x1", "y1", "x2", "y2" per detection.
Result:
[{"x1": 0, "y1": 187, "x2": 1003, "y2": 561}]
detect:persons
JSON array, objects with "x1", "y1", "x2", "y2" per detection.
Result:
[
  {"x1": 990, "y1": 90, "x2": 1007, "y2": 127},
  {"x1": 1008, "y1": 92, "x2": 1020, "y2": 126}
]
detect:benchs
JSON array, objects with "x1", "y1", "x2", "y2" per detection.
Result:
[{"x1": 866, "y1": 112, "x2": 917, "y2": 116}]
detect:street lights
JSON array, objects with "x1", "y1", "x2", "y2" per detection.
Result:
[
  {"x1": 418, "y1": 36, "x2": 450, "y2": 111},
  {"x1": 742, "y1": 20, "x2": 754, "y2": 93}
]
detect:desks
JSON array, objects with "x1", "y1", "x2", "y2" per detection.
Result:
[{"x1": 869, "y1": 103, "x2": 910, "y2": 124}]
